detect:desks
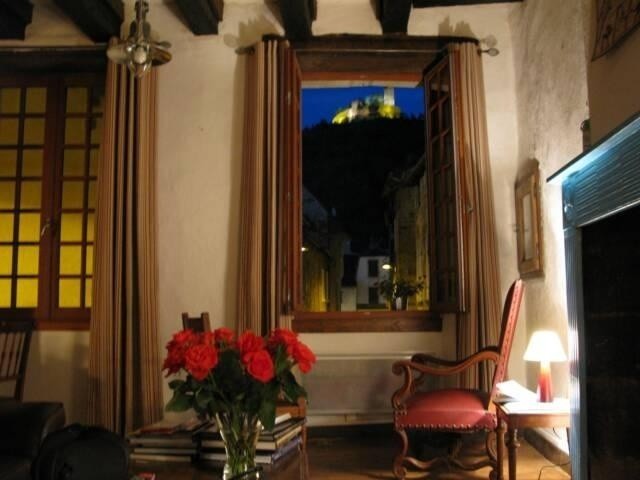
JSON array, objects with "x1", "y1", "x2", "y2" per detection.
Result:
[{"x1": 123, "y1": 442, "x2": 301, "y2": 480}]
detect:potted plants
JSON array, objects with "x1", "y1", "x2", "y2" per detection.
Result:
[{"x1": 379, "y1": 277, "x2": 425, "y2": 311}]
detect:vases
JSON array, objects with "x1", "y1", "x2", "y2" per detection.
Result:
[{"x1": 215, "y1": 411, "x2": 265, "y2": 480}]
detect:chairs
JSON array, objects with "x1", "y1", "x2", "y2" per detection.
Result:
[
  {"x1": 390, "y1": 276, "x2": 526, "y2": 480},
  {"x1": 181, "y1": 312, "x2": 307, "y2": 451},
  {"x1": 0, "y1": 318, "x2": 34, "y2": 400}
]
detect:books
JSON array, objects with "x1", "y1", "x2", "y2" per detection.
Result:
[{"x1": 127, "y1": 413, "x2": 306, "y2": 464}]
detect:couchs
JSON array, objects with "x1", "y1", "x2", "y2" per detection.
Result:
[{"x1": 0, "y1": 398, "x2": 67, "y2": 480}]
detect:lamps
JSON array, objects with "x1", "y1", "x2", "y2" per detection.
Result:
[
  {"x1": 106, "y1": 0, "x2": 173, "y2": 78},
  {"x1": 523, "y1": 330, "x2": 568, "y2": 403}
]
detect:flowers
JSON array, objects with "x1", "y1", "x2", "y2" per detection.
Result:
[{"x1": 160, "y1": 326, "x2": 317, "y2": 477}]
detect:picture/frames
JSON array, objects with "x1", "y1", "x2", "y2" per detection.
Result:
[{"x1": 514, "y1": 173, "x2": 543, "y2": 276}]
